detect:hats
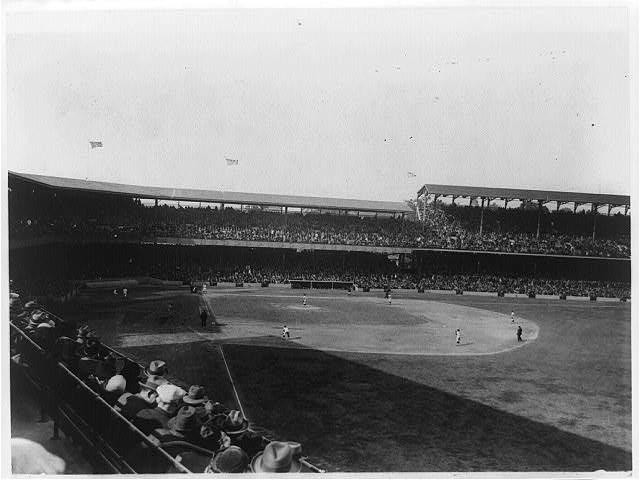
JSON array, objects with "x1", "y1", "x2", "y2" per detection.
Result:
[
  {"x1": 185, "y1": 423, "x2": 232, "y2": 453},
  {"x1": 183, "y1": 385, "x2": 209, "y2": 404},
  {"x1": 222, "y1": 410, "x2": 249, "y2": 434},
  {"x1": 10, "y1": 435, "x2": 67, "y2": 473},
  {"x1": 210, "y1": 446, "x2": 249, "y2": 473},
  {"x1": 21, "y1": 289, "x2": 134, "y2": 378},
  {"x1": 167, "y1": 407, "x2": 203, "y2": 431},
  {"x1": 250, "y1": 441, "x2": 303, "y2": 473},
  {"x1": 157, "y1": 384, "x2": 186, "y2": 404},
  {"x1": 229, "y1": 429, "x2": 266, "y2": 459},
  {"x1": 144, "y1": 360, "x2": 168, "y2": 378},
  {"x1": 288, "y1": 441, "x2": 308, "y2": 462},
  {"x1": 138, "y1": 375, "x2": 168, "y2": 392}
]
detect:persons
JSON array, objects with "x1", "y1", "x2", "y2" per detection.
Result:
[
  {"x1": 10, "y1": 292, "x2": 308, "y2": 472},
  {"x1": 455, "y1": 328, "x2": 464, "y2": 346},
  {"x1": 516, "y1": 326, "x2": 522, "y2": 344},
  {"x1": 388, "y1": 293, "x2": 392, "y2": 304},
  {"x1": 511, "y1": 310, "x2": 515, "y2": 324},
  {"x1": 10, "y1": 203, "x2": 631, "y2": 302}
]
